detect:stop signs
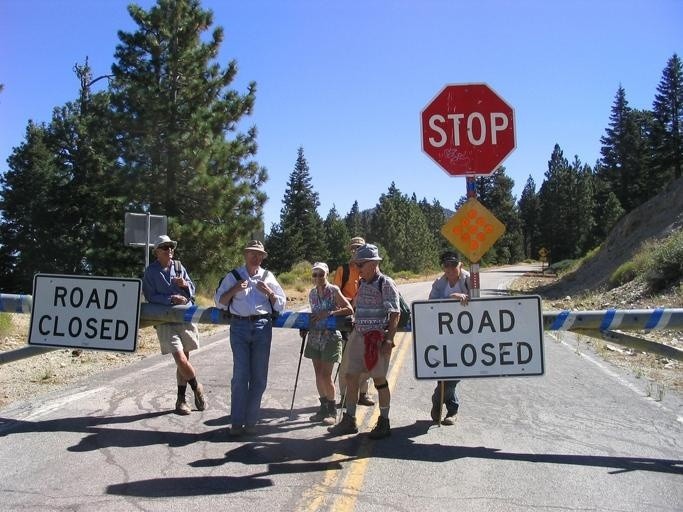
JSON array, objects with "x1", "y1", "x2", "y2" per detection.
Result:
[{"x1": 419, "y1": 82, "x2": 518, "y2": 180}]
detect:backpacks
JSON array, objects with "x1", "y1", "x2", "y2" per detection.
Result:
[{"x1": 376, "y1": 274, "x2": 412, "y2": 329}]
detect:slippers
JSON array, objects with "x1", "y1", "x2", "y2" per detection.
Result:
[{"x1": 369, "y1": 414, "x2": 392, "y2": 441}]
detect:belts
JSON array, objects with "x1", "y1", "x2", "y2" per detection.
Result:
[{"x1": 228, "y1": 313, "x2": 271, "y2": 321}]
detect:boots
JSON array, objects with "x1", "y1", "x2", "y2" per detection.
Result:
[
  {"x1": 326, "y1": 413, "x2": 358, "y2": 435},
  {"x1": 309, "y1": 396, "x2": 328, "y2": 422},
  {"x1": 323, "y1": 399, "x2": 337, "y2": 425}
]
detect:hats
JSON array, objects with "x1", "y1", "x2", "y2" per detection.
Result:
[
  {"x1": 312, "y1": 262, "x2": 329, "y2": 274},
  {"x1": 153, "y1": 234, "x2": 178, "y2": 252},
  {"x1": 352, "y1": 243, "x2": 385, "y2": 262},
  {"x1": 439, "y1": 252, "x2": 461, "y2": 265},
  {"x1": 243, "y1": 240, "x2": 270, "y2": 258},
  {"x1": 349, "y1": 236, "x2": 366, "y2": 247}
]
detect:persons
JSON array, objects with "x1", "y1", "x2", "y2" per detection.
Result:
[
  {"x1": 327, "y1": 244, "x2": 402, "y2": 438},
  {"x1": 428, "y1": 251, "x2": 471, "y2": 425},
  {"x1": 299, "y1": 261, "x2": 354, "y2": 425},
  {"x1": 333, "y1": 237, "x2": 375, "y2": 408},
  {"x1": 142, "y1": 235, "x2": 207, "y2": 415},
  {"x1": 213, "y1": 240, "x2": 287, "y2": 436}
]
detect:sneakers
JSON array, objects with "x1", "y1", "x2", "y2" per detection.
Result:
[
  {"x1": 359, "y1": 393, "x2": 376, "y2": 406},
  {"x1": 230, "y1": 425, "x2": 243, "y2": 436},
  {"x1": 245, "y1": 424, "x2": 259, "y2": 436},
  {"x1": 174, "y1": 397, "x2": 196, "y2": 416},
  {"x1": 443, "y1": 411, "x2": 459, "y2": 425},
  {"x1": 430, "y1": 394, "x2": 442, "y2": 421},
  {"x1": 191, "y1": 382, "x2": 208, "y2": 410}
]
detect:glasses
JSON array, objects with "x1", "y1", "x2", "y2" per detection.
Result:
[
  {"x1": 354, "y1": 260, "x2": 369, "y2": 268},
  {"x1": 158, "y1": 245, "x2": 176, "y2": 251}
]
